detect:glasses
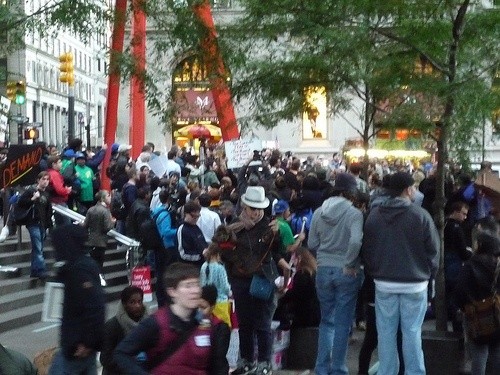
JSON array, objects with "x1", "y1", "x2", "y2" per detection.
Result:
[{"x1": 189, "y1": 213, "x2": 202, "y2": 219}]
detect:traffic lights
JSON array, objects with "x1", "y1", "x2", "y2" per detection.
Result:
[
  {"x1": 59, "y1": 52, "x2": 73, "y2": 83},
  {"x1": 15, "y1": 80, "x2": 27, "y2": 104},
  {"x1": 24, "y1": 129, "x2": 39, "y2": 140}
]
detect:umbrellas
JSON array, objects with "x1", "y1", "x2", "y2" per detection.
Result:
[{"x1": 174, "y1": 124, "x2": 222, "y2": 137}]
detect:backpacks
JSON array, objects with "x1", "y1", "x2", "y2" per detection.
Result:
[{"x1": 140, "y1": 210, "x2": 170, "y2": 249}]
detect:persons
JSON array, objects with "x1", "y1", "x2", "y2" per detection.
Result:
[
  {"x1": 220, "y1": 185, "x2": 292, "y2": 375},
  {"x1": 277, "y1": 247, "x2": 320, "y2": 375},
  {"x1": 0, "y1": 139, "x2": 132, "y2": 278},
  {"x1": 307, "y1": 172, "x2": 362, "y2": 375},
  {"x1": 47, "y1": 223, "x2": 104, "y2": 375},
  {"x1": 271, "y1": 148, "x2": 433, "y2": 252},
  {"x1": 419, "y1": 162, "x2": 500, "y2": 375},
  {"x1": 121, "y1": 142, "x2": 270, "y2": 306},
  {"x1": 100, "y1": 263, "x2": 231, "y2": 375},
  {"x1": 360, "y1": 171, "x2": 440, "y2": 375}
]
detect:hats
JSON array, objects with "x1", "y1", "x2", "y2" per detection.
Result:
[
  {"x1": 334, "y1": 174, "x2": 355, "y2": 192},
  {"x1": 272, "y1": 200, "x2": 288, "y2": 214},
  {"x1": 112, "y1": 143, "x2": 119, "y2": 154},
  {"x1": 63, "y1": 149, "x2": 77, "y2": 158},
  {"x1": 240, "y1": 186, "x2": 269, "y2": 209},
  {"x1": 118, "y1": 144, "x2": 133, "y2": 154},
  {"x1": 75, "y1": 153, "x2": 85, "y2": 159}
]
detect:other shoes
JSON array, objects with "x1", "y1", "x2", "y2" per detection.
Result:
[
  {"x1": 29, "y1": 269, "x2": 50, "y2": 281},
  {"x1": 232, "y1": 359, "x2": 257, "y2": 375},
  {"x1": 244, "y1": 360, "x2": 272, "y2": 375}
]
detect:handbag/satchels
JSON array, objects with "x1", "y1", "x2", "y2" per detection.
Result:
[
  {"x1": 462, "y1": 294, "x2": 500, "y2": 343},
  {"x1": 9, "y1": 186, "x2": 41, "y2": 226},
  {"x1": 214, "y1": 303, "x2": 232, "y2": 329},
  {"x1": 249, "y1": 273, "x2": 276, "y2": 302}
]
